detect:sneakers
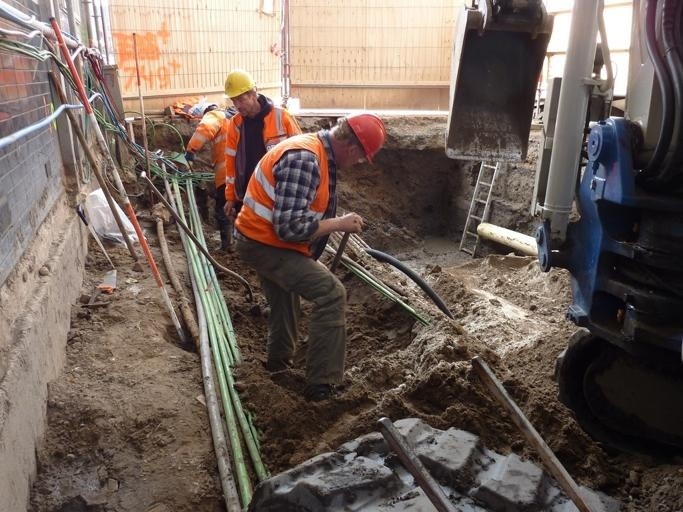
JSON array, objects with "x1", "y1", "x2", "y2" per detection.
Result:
[{"x1": 303, "y1": 386, "x2": 329, "y2": 402}]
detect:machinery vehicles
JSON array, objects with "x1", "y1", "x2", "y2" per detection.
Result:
[{"x1": 438, "y1": 1, "x2": 681, "y2": 432}]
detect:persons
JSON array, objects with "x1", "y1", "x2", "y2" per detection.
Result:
[
  {"x1": 228, "y1": 114, "x2": 386, "y2": 394},
  {"x1": 220, "y1": 69, "x2": 308, "y2": 220},
  {"x1": 184, "y1": 99, "x2": 234, "y2": 255}
]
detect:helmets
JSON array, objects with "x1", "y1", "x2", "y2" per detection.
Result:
[
  {"x1": 346, "y1": 114, "x2": 385, "y2": 165},
  {"x1": 224, "y1": 72, "x2": 254, "y2": 99}
]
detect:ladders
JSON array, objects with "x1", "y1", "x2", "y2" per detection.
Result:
[{"x1": 459, "y1": 160, "x2": 502, "y2": 259}]
